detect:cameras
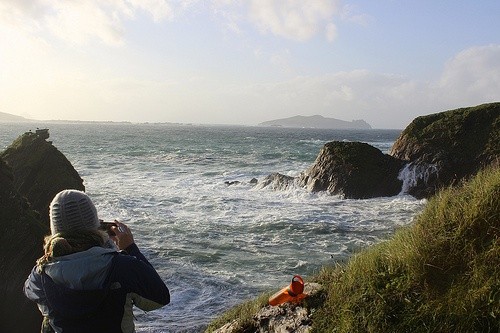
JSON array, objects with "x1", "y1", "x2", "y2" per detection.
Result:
[{"x1": 99, "y1": 221, "x2": 117, "y2": 235}]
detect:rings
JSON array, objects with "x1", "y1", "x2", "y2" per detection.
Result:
[{"x1": 117, "y1": 226, "x2": 125, "y2": 233}]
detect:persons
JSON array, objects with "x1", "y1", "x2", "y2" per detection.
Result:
[{"x1": 23, "y1": 189, "x2": 170, "y2": 333}]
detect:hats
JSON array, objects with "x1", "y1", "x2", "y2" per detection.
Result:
[{"x1": 49, "y1": 190, "x2": 99, "y2": 237}]
